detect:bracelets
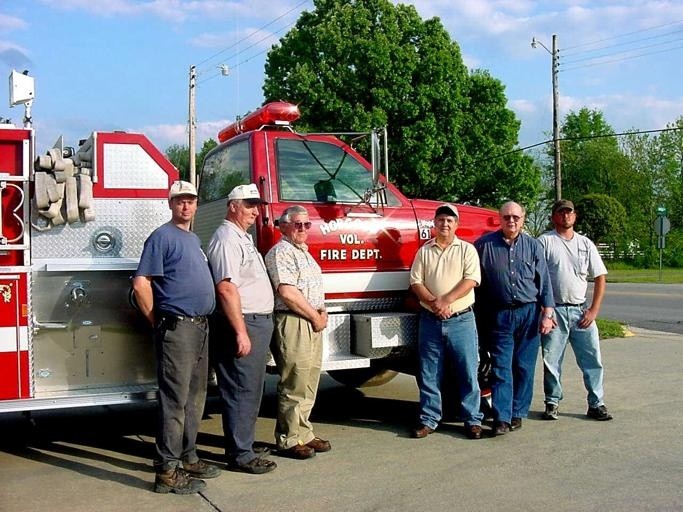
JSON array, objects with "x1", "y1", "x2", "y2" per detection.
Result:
[{"x1": 542, "y1": 310, "x2": 555, "y2": 319}]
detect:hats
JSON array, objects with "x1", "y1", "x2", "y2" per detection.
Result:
[
  {"x1": 227, "y1": 183, "x2": 268, "y2": 205},
  {"x1": 552, "y1": 200, "x2": 574, "y2": 215},
  {"x1": 169, "y1": 180, "x2": 198, "y2": 200},
  {"x1": 435, "y1": 203, "x2": 458, "y2": 217}
]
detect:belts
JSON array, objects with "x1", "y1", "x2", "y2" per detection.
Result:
[
  {"x1": 448, "y1": 308, "x2": 471, "y2": 319},
  {"x1": 176, "y1": 314, "x2": 206, "y2": 322}
]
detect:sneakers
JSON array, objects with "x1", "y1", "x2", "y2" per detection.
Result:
[
  {"x1": 156, "y1": 468, "x2": 206, "y2": 494},
  {"x1": 587, "y1": 406, "x2": 613, "y2": 420},
  {"x1": 544, "y1": 404, "x2": 559, "y2": 420},
  {"x1": 180, "y1": 457, "x2": 222, "y2": 478}
]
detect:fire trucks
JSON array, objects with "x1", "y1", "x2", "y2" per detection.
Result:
[{"x1": 0, "y1": 95, "x2": 506, "y2": 422}]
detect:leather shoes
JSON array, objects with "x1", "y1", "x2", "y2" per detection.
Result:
[
  {"x1": 413, "y1": 425, "x2": 436, "y2": 438},
  {"x1": 277, "y1": 437, "x2": 331, "y2": 458},
  {"x1": 465, "y1": 416, "x2": 522, "y2": 438},
  {"x1": 225, "y1": 445, "x2": 277, "y2": 473}
]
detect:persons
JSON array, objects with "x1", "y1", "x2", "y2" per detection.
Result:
[
  {"x1": 408, "y1": 203, "x2": 485, "y2": 438},
  {"x1": 472, "y1": 200, "x2": 556, "y2": 435},
  {"x1": 132, "y1": 179, "x2": 221, "y2": 497},
  {"x1": 534, "y1": 199, "x2": 610, "y2": 419},
  {"x1": 206, "y1": 182, "x2": 276, "y2": 476},
  {"x1": 262, "y1": 206, "x2": 331, "y2": 459}
]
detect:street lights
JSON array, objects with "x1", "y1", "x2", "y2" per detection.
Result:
[
  {"x1": 529, "y1": 35, "x2": 563, "y2": 201},
  {"x1": 189, "y1": 64, "x2": 232, "y2": 186}
]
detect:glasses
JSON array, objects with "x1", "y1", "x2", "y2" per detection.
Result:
[
  {"x1": 291, "y1": 221, "x2": 311, "y2": 229},
  {"x1": 503, "y1": 215, "x2": 521, "y2": 220}
]
